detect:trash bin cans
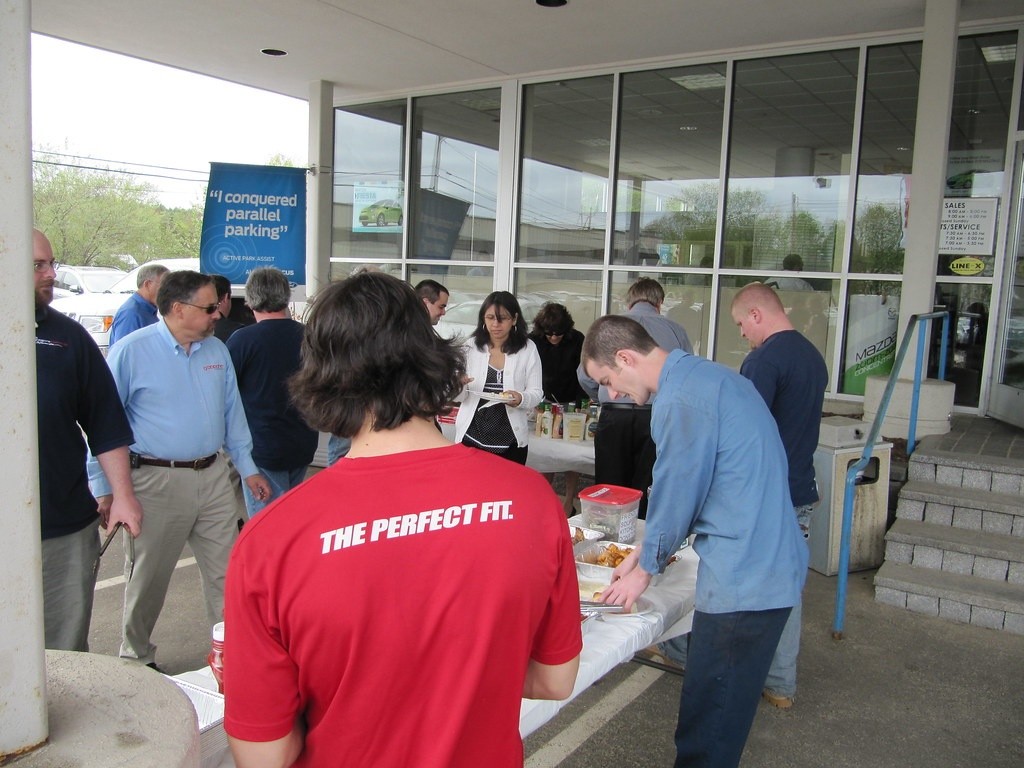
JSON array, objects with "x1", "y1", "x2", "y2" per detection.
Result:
[{"x1": 811, "y1": 418, "x2": 893, "y2": 577}]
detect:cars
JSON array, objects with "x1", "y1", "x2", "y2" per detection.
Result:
[
  {"x1": 432, "y1": 289, "x2": 703, "y2": 347},
  {"x1": 359, "y1": 199, "x2": 402, "y2": 227},
  {"x1": 957, "y1": 317, "x2": 1024, "y2": 352}
]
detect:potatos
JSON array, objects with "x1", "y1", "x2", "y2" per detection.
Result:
[{"x1": 596, "y1": 544, "x2": 633, "y2": 567}]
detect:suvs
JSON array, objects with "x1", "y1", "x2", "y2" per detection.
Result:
[
  {"x1": 52, "y1": 263, "x2": 129, "y2": 297},
  {"x1": 48, "y1": 257, "x2": 200, "y2": 357}
]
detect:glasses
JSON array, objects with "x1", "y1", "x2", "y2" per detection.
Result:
[
  {"x1": 484, "y1": 315, "x2": 512, "y2": 324},
  {"x1": 175, "y1": 299, "x2": 221, "y2": 314}
]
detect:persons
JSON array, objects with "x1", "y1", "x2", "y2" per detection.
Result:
[
  {"x1": 90, "y1": 271, "x2": 272, "y2": 676},
  {"x1": 766, "y1": 252, "x2": 813, "y2": 291},
  {"x1": 582, "y1": 315, "x2": 801, "y2": 768},
  {"x1": 224, "y1": 274, "x2": 581, "y2": 768},
  {"x1": 685, "y1": 257, "x2": 712, "y2": 287},
  {"x1": 528, "y1": 303, "x2": 591, "y2": 403},
  {"x1": 108, "y1": 265, "x2": 318, "y2": 518},
  {"x1": 449, "y1": 291, "x2": 545, "y2": 466},
  {"x1": 328, "y1": 280, "x2": 467, "y2": 464},
  {"x1": 578, "y1": 279, "x2": 693, "y2": 520},
  {"x1": 648, "y1": 281, "x2": 828, "y2": 711},
  {"x1": 33, "y1": 227, "x2": 143, "y2": 654}
]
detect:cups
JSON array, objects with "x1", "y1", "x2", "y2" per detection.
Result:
[{"x1": 562, "y1": 412, "x2": 587, "y2": 442}]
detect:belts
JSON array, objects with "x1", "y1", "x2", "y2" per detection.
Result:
[
  {"x1": 601, "y1": 402, "x2": 651, "y2": 410},
  {"x1": 140, "y1": 452, "x2": 220, "y2": 470}
]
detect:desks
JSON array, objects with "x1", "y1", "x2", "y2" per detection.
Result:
[
  {"x1": 524, "y1": 416, "x2": 595, "y2": 476},
  {"x1": 171, "y1": 511, "x2": 702, "y2": 768}
]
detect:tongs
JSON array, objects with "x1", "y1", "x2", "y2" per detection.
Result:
[
  {"x1": 92, "y1": 522, "x2": 135, "y2": 582},
  {"x1": 580, "y1": 600, "x2": 623, "y2": 612}
]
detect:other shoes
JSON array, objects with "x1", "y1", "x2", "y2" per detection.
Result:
[{"x1": 762, "y1": 687, "x2": 794, "y2": 708}]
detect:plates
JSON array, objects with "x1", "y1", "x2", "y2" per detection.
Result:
[
  {"x1": 601, "y1": 595, "x2": 655, "y2": 616},
  {"x1": 467, "y1": 390, "x2": 516, "y2": 402}
]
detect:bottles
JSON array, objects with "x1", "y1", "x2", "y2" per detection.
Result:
[
  {"x1": 535, "y1": 404, "x2": 552, "y2": 437},
  {"x1": 568, "y1": 402, "x2": 575, "y2": 412},
  {"x1": 206, "y1": 621, "x2": 225, "y2": 694},
  {"x1": 552, "y1": 403, "x2": 563, "y2": 438},
  {"x1": 580, "y1": 399, "x2": 598, "y2": 442}
]
